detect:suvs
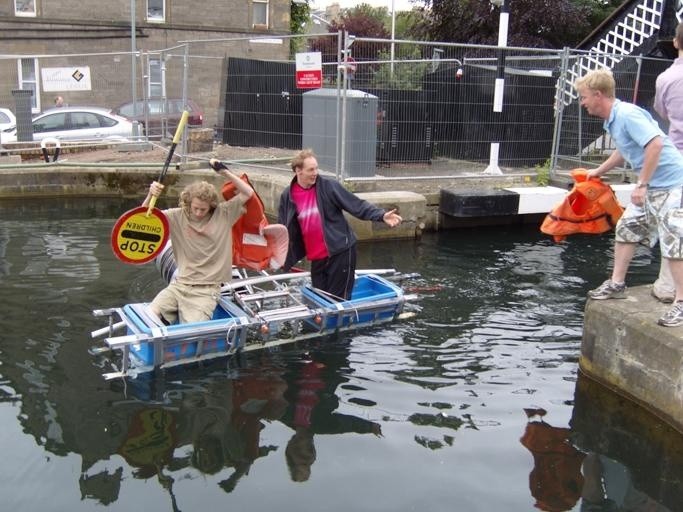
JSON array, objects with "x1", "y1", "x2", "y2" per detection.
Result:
[{"x1": 111, "y1": 97, "x2": 203, "y2": 140}]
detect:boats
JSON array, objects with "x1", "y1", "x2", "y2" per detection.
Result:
[
  {"x1": 88, "y1": 266, "x2": 425, "y2": 380},
  {"x1": 101, "y1": 321, "x2": 415, "y2": 413}
]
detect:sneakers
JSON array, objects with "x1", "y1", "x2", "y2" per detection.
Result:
[
  {"x1": 657, "y1": 302, "x2": 683, "y2": 328},
  {"x1": 650, "y1": 286, "x2": 675, "y2": 304},
  {"x1": 586, "y1": 279, "x2": 625, "y2": 299}
]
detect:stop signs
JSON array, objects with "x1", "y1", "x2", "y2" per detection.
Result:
[{"x1": 111, "y1": 208, "x2": 169, "y2": 266}]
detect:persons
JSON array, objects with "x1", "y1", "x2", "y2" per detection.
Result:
[
  {"x1": 266, "y1": 328, "x2": 386, "y2": 473},
  {"x1": 141, "y1": 158, "x2": 254, "y2": 325},
  {"x1": 157, "y1": 365, "x2": 248, "y2": 493},
  {"x1": 651, "y1": 23, "x2": 683, "y2": 303},
  {"x1": 278, "y1": 149, "x2": 403, "y2": 304},
  {"x1": 55, "y1": 96, "x2": 65, "y2": 110},
  {"x1": 575, "y1": 69, "x2": 683, "y2": 327}
]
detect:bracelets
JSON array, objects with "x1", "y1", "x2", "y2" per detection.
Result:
[{"x1": 636, "y1": 181, "x2": 650, "y2": 188}]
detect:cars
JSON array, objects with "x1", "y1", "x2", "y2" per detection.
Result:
[
  {"x1": 0, "y1": 107, "x2": 17, "y2": 144},
  {"x1": 4, "y1": 107, "x2": 142, "y2": 144}
]
